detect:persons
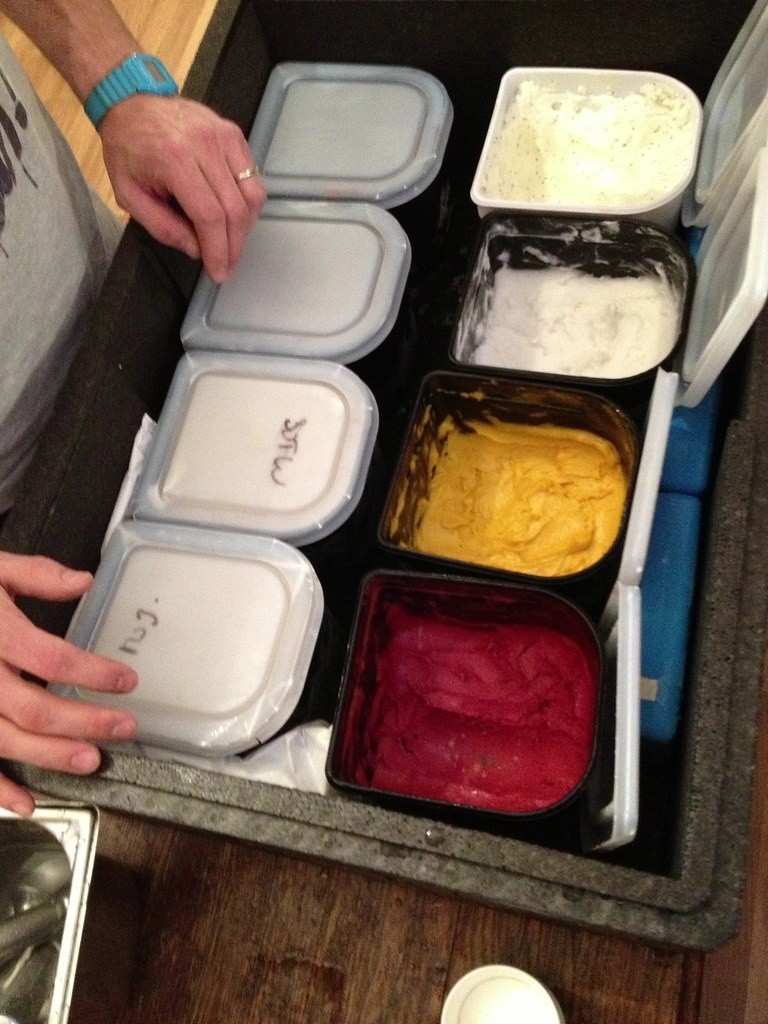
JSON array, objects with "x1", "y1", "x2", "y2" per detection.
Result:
[{"x1": 0, "y1": 0, "x2": 266, "y2": 818}]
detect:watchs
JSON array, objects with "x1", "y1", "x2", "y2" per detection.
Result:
[{"x1": 84, "y1": 54, "x2": 179, "y2": 129}]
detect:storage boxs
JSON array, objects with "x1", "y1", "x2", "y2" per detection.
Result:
[{"x1": 68, "y1": 0, "x2": 768, "y2": 855}]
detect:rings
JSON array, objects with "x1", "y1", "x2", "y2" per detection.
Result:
[{"x1": 234, "y1": 167, "x2": 257, "y2": 184}]
